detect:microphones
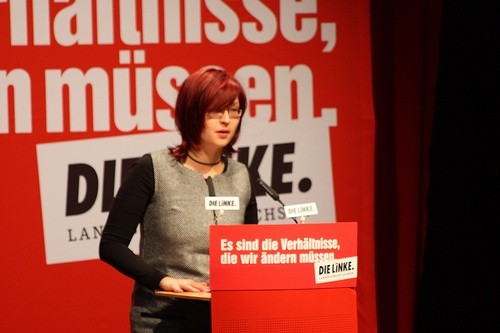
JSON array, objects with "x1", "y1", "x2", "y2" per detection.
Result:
[
  {"x1": 204, "y1": 176, "x2": 218, "y2": 226},
  {"x1": 255, "y1": 178, "x2": 298, "y2": 224}
]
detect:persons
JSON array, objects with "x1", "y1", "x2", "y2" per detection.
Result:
[{"x1": 99, "y1": 67, "x2": 259, "y2": 333}]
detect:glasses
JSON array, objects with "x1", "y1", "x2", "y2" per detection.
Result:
[{"x1": 207, "y1": 106, "x2": 242, "y2": 118}]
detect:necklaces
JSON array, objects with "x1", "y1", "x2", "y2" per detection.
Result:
[{"x1": 185, "y1": 152, "x2": 223, "y2": 166}]
